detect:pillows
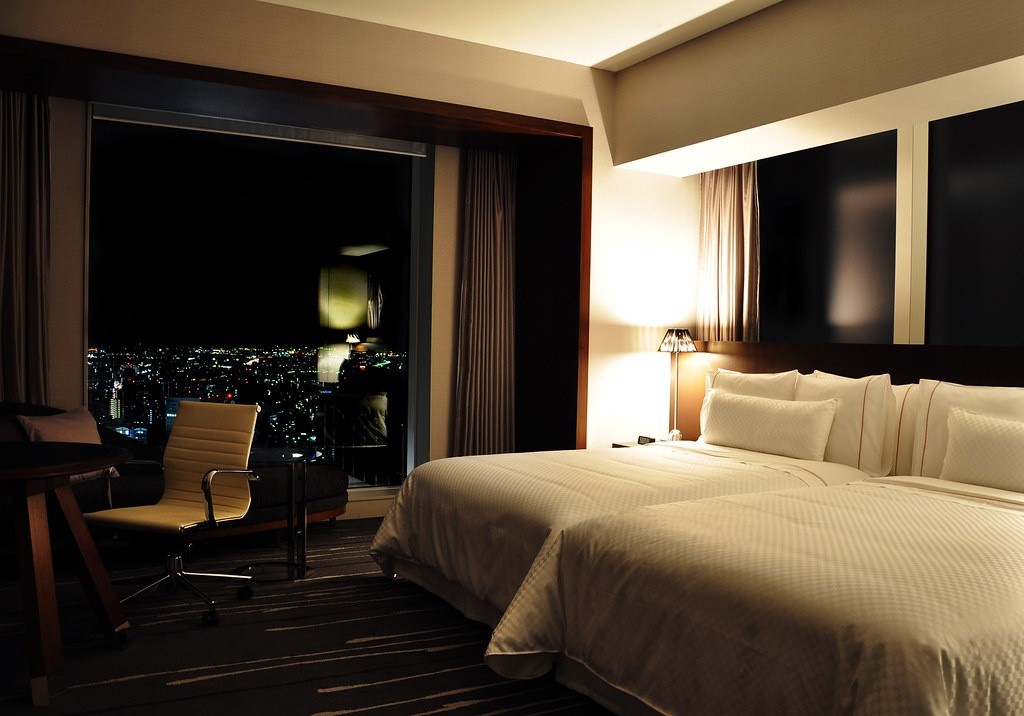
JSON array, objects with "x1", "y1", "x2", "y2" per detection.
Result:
[
  {"x1": 16, "y1": 406, "x2": 102, "y2": 444},
  {"x1": 914, "y1": 378, "x2": 1024, "y2": 491},
  {"x1": 699, "y1": 389, "x2": 839, "y2": 462},
  {"x1": 710, "y1": 370, "x2": 797, "y2": 400},
  {"x1": 796, "y1": 369, "x2": 898, "y2": 475}
]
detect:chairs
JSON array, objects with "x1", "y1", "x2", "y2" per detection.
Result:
[{"x1": 81, "y1": 401, "x2": 261, "y2": 626}]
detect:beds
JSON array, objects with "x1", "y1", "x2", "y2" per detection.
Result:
[
  {"x1": 484, "y1": 379, "x2": 1024, "y2": 716},
  {"x1": 370, "y1": 366, "x2": 919, "y2": 631}
]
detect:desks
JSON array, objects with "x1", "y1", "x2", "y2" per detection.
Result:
[{"x1": 1, "y1": 440, "x2": 129, "y2": 677}]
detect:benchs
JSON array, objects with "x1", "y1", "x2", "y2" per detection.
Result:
[{"x1": 0, "y1": 402, "x2": 349, "y2": 541}]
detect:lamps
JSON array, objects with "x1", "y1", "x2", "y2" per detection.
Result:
[{"x1": 657, "y1": 328, "x2": 698, "y2": 441}]
